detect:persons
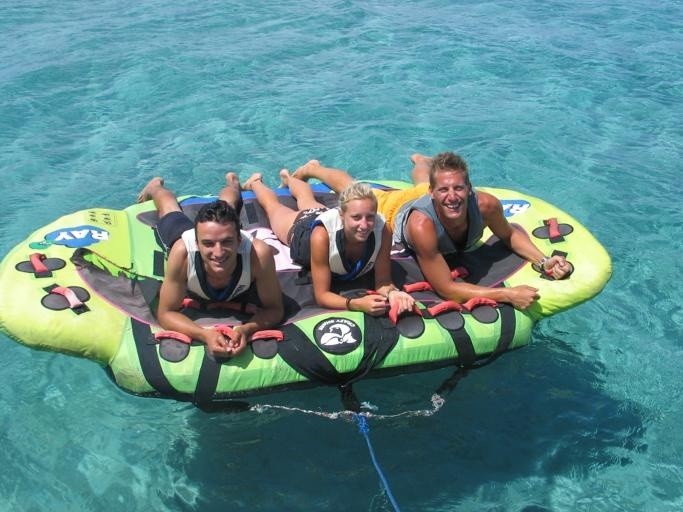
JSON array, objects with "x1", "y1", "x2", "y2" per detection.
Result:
[
  {"x1": 292, "y1": 152, "x2": 572, "y2": 309},
  {"x1": 137, "y1": 170, "x2": 287, "y2": 358},
  {"x1": 243, "y1": 170, "x2": 415, "y2": 317}
]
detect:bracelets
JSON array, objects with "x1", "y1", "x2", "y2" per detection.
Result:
[
  {"x1": 537, "y1": 255, "x2": 550, "y2": 266},
  {"x1": 345, "y1": 296, "x2": 352, "y2": 311},
  {"x1": 385, "y1": 287, "x2": 400, "y2": 298}
]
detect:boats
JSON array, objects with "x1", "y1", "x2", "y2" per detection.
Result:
[{"x1": 0, "y1": 180, "x2": 613, "y2": 399}]
